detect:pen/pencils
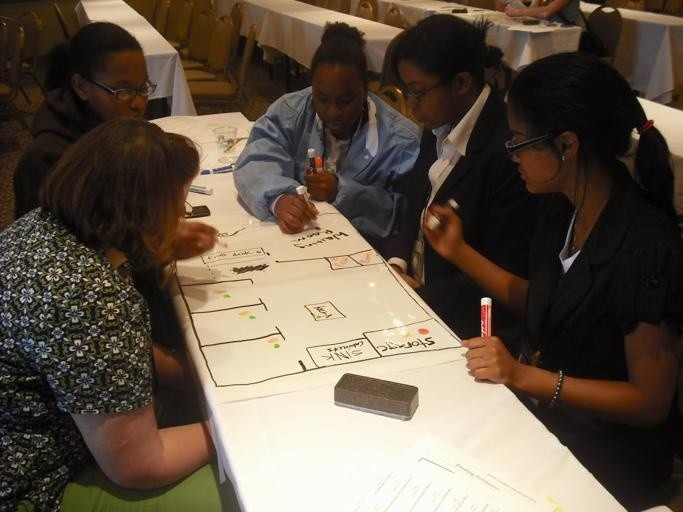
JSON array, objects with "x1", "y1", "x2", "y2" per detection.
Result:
[
  {"x1": 200, "y1": 165, "x2": 234, "y2": 175},
  {"x1": 296, "y1": 184, "x2": 321, "y2": 232},
  {"x1": 479, "y1": 296, "x2": 492, "y2": 340},
  {"x1": 190, "y1": 184, "x2": 212, "y2": 197},
  {"x1": 306, "y1": 149, "x2": 324, "y2": 177},
  {"x1": 425, "y1": 198, "x2": 459, "y2": 234}
]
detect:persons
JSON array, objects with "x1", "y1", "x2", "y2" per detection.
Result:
[
  {"x1": 497, "y1": 0, "x2": 587, "y2": 35},
  {"x1": 423, "y1": 51, "x2": 682, "y2": 512},
  {"x1": 234, "y1": 22, "x2": 422, "y2": 255},
  {"x1": 0, "y1": 117, "x2": 239, "y2": 512},
  {"x1": 13, "y1": 23, "x2": 218, "y2": 348},
  {"x1": 381, "y1": 14, "x2": 544, "y2": 338}
]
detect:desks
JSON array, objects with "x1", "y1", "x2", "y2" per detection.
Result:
[
  {"x1": 147, "y1": 111, "x2": 628, "y2": 511},
  {"x1": 348, "y1": 1, "x2": 584, "y2": 72},
  {"x1": 617, "y1": 96, "x2": 681, "y2": 217},
  {"x1": 207, "y1": 0, "x2": 405, "y2": 94},
  {"x1": 77, "y1": 0, "x2": 196, "y2": 114},
  {"x1": 495, "y1": 0, "x2": 682, "y2": 105}
]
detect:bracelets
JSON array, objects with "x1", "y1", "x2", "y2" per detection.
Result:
[{"x1": 548, "y1": 370, "x2": 563, "y2": 411}]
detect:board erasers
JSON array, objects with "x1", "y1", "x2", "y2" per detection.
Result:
[{"x1": 335, "y1": 372, "x2": 420, "y2": 421}]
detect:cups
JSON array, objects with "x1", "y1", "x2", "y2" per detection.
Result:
[
  {"x1": 538, "y1": 12, "x2": 550, "y2": 26},
  {"x1": 213, "y1": 126, "x2": 237, "y2": 163}
]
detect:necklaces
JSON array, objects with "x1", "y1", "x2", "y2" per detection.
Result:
[{"x1": 570, "y1": 217, "x2": 595, "y2": 256}]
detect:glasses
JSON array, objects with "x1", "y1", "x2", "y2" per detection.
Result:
[
  {"x1": 88, "y1": 77, "x2": 159, "y2": 100},
  {"x1": 402, "y1": 80, "x2": 446, "y2": 99},
  {"x1": 504, "y1": 133, "x2": 558, "y2": 157}
]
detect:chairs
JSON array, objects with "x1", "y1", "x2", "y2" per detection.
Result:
[
  {"x1": 354, "y1": 1, "x2": 375, "y2": 21},
  {"x1": 567, "y1": 4, "x2": 623, "y2": 65},
  {"x1": 618, "y1": 1, "x2": 682, "y2": 16},
  {"x1": 377, "y1": 86, "x2": 407, "y2": 118},
  {"x1": 152, "y1": 0, "x2": 197, "y2": 60},
  {"x1": 382, "y1": 5, "x2": 404, "y2": 28},
  {"x1": 180, "y1": 1, "x2": 257, "y2": 117}
]
